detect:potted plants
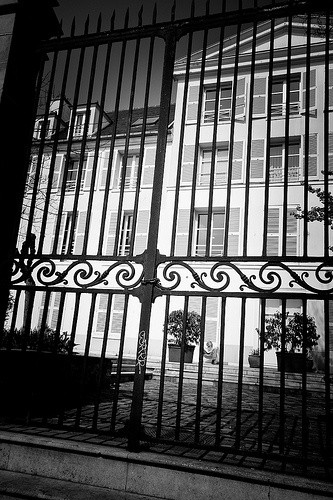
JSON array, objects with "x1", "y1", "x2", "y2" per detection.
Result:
[
  {"x1": 163, "y1": 310, "x2": 201, "y2": 363},
  {"x1": 256, "y1": 311, "x2": 320, "y2": 373},
  {"x1": 248, "y1": 317, "x2": 276, "y2": 368}
]
